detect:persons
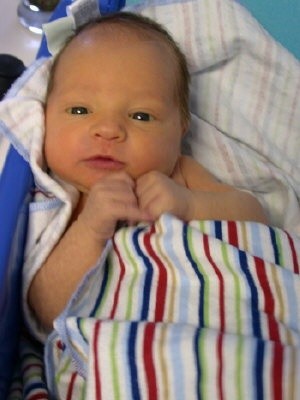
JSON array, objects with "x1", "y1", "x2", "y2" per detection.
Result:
[{"x1": 23, "y1": 13, "x2": 267, "y2": 349}]
